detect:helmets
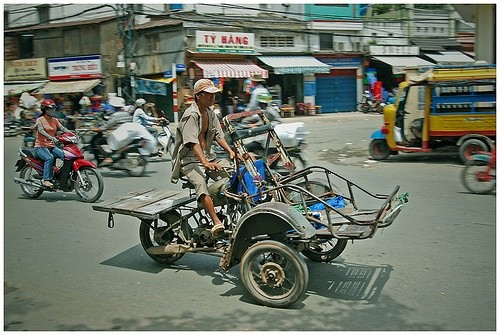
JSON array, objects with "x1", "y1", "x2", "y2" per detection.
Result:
[{"x1": 41, "y1": 98, "x2": 57, "y2": 108}]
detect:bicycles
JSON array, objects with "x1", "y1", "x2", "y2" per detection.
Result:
[{"x1": 208, "y1": 120, "x2": 312, "y2": 186}]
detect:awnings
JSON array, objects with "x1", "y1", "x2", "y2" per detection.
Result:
[
  {"x1": 255, "y1": 57, "x2": 331, "y2": 75},
  {"x1": 424, "y1": 53, "x2": 476, "y2": 66},
  {"x1": 193, "y1": 58, "x2": 270, "y2": 80},
  {"x1": 374, "y1": 55, "x2": 436, "y2": 75},
  {"x1": 32, "y1": 79, "x2": 100, "y2": 96},
  {"x1": 4, "y1": 80, "x2": 50, "y2": 98}
]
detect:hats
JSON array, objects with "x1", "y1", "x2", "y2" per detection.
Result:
[
  {"x1": 193, "y1": 79, "x2": 222, "y2": 95},
  {"x1": 250, "y1": 74, "x2": 266, "y2": 81},
  {"x1": 109, "y1": 97, "x2": 126, "y2": 108},
  {"x1": 135, "y1": 98, "x2": 146, "y2": 107}
]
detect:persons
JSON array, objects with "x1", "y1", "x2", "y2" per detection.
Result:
[
  {"x1": 71, "y1": 111, "x2": 83, "y2": 130},
  {"x1": 20, "y1": 93, "x2": 43, "y2": 146},
  {"x1": 35, "y1": 100, "x2": 82, "y2": 189},
  {"x1": 133, "y1": 99, "x2": 170, "y2": 156},
  {"x1": 242, "y1": 94, "x2": 282, "y2": 166},
  {"x1": 226, "y1": 89, "x2": 235, "y2": 107},
  {"x1": 89, "y1": 96, "x2": 135, "y2": 168},
  {"x1": 242, "y1": 73, "x2": 272, "y2": 114},
  {"x1": 171, "y1": 79, "x2": 246, "y2": 236}
]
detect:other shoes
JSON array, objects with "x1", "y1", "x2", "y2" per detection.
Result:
[
  {"x1": 102, "y1": 158, "x2": 114, "y2": 163},
  {"x1": 211, "y1": 224, "x2": 225, "y2": 238}
]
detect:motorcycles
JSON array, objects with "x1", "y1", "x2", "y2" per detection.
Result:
[
  {"x1": 142, "y1": 111, "x2": 179, "y2": 160},
  {"x1": 77, "y1": 127, "x2": 148, "y2": 178},
  {"x1": 367, "y1": 63, "x2": 496, "y2": 165},
  {"x1": 357, "y1": 91, "x2": 391, "y2": 115},
  {"x1": 14, "y1": 134, "x2": 104, "y2": 202},
  {"x1": 458, "y1": 154, "x2": 495, "y2": 194}
]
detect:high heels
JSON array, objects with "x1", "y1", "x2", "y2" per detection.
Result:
[{"x1": 41, "y1": 181, "x2": 53, "y2": 187}]
